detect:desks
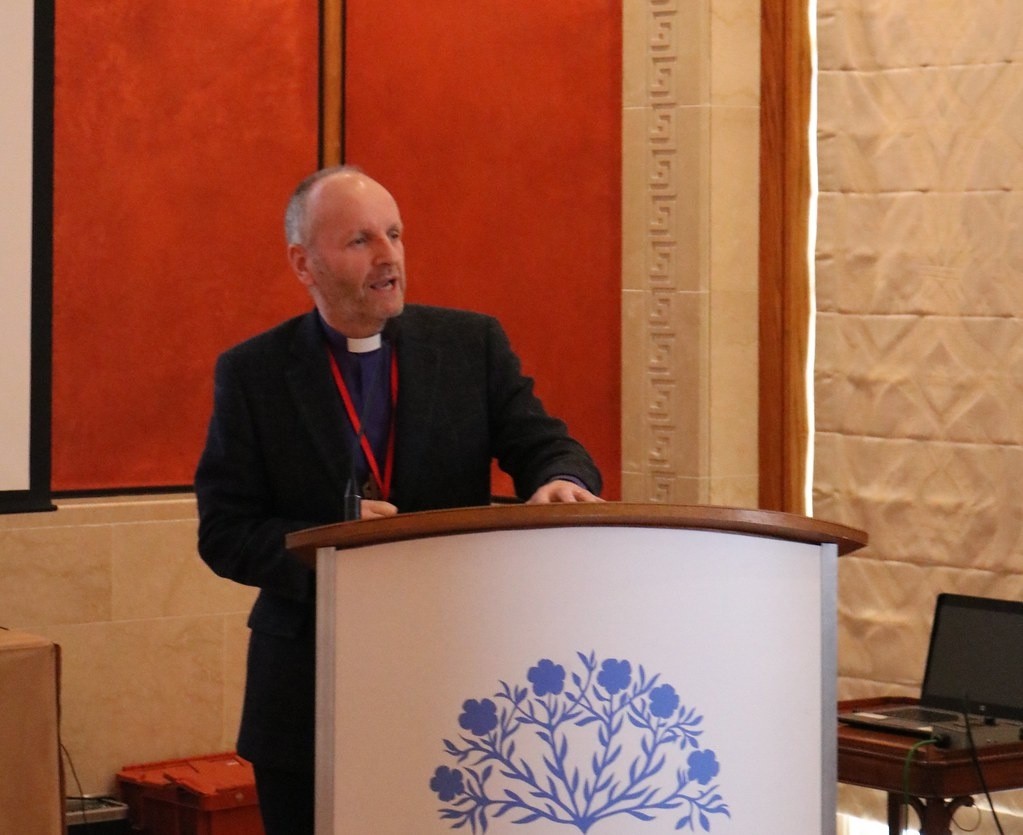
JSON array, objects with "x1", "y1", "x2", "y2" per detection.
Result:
[
  {"x1": 837, "y1": 696, "x2": 1023, "y2": 835},
  {"x1": 0, "y1": 627, "x2": 66, "y2": 835}
]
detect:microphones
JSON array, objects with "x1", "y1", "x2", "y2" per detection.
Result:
[{"x1": 344, "y1": 317, "x2": 400, "y2": 521}]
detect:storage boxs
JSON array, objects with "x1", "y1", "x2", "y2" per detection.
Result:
[{"x1": 114, "y1": 753, "x2": 266, "y2": 835}]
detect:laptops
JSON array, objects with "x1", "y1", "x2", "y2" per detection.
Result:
[{"x1": 837, "y1": 594, "x2": 1023, "y2": 742}]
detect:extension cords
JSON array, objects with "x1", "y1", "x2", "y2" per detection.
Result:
[{"x1": 930, "y1": 720, "x2": 1021, "y2": 752}]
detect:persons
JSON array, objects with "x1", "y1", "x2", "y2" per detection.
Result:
[{"x1": 195, "y1": 164, "x2": 613, "y2": 835}]
